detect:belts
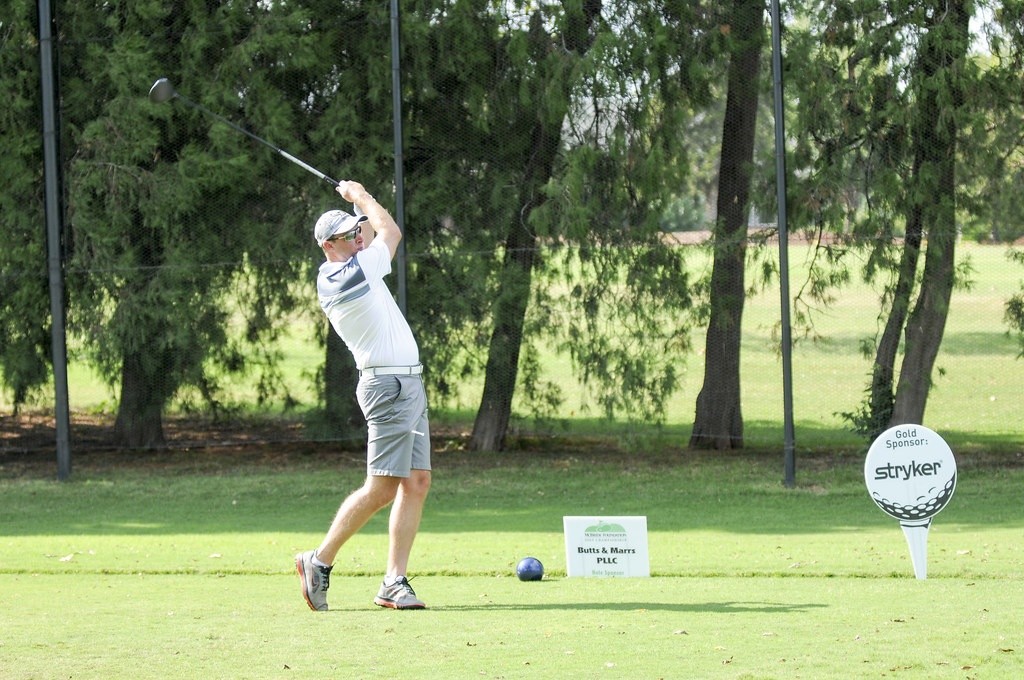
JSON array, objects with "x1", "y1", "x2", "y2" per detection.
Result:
[{"x1": 361, "y1": 363, "x2": 423, "y2": 376}]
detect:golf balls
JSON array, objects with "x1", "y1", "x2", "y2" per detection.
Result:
[{"x1": 989, "y1": 395, "x2": 996, "y2": 401}]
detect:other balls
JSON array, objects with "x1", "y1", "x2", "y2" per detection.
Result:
[{"x1": 517, "y1": 557, "x2": 543, "y2": 581}]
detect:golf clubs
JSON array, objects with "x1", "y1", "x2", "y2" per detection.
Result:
[{"x1": 148, "y1": 78, "x2": 340, "y2": 187}]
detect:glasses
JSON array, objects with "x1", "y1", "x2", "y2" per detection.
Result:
[{"x1": 326, "y1": 226, "x2": 361, "y2": 241}]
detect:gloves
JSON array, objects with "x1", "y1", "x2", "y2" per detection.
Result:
[{"x1": 353, "y1": 200, "x2": 366, "y2": 217}]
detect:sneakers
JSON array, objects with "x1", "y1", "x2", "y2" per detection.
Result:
[
  {"x1": 374, "y1": 574, "x2": 425, "y2": 609},
  {"x1": 297, "y1": 549, "x2": 336, "y2": 611}
]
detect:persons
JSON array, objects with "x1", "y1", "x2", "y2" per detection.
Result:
[{"x1": 295, "y1": 180, "x2": 432, "y2": 610}]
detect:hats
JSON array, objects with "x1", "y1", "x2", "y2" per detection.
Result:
[{"x1": 314, "y1": 210, "x2": 368, "y2": 247}]
